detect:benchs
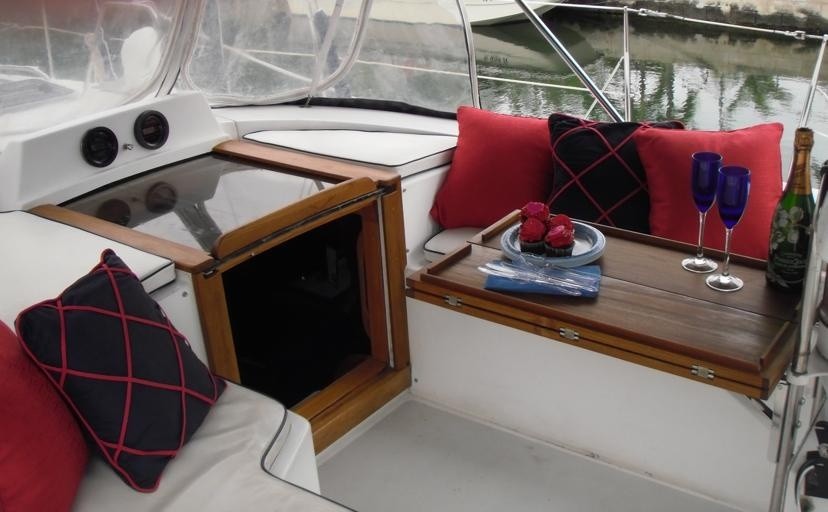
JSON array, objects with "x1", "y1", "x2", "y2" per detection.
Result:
[{"x1": 402, "y1": 221, "x2": 819, "y2": 512}]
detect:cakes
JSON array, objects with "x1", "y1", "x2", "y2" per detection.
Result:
[
  {"x1": 519, "y1": 218, "x2": 546, "y2": 255},
  {"x1": 543, "y1": 225, "x2": 576, "y2": 257},
  {"x1": 545, "y1": 213, "x2": 574, "y2": 232},
  {"x1": 520, "y1": 201, "x2": 550, "y2": 223}
]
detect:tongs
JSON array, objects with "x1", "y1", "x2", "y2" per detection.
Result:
[{"x1": 477, "y1": 254, "x2": 601, "y2": 297}]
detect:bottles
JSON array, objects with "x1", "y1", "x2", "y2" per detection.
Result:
[{"x1": 764, "y1": 126, "x2": 815, "y2": 296}]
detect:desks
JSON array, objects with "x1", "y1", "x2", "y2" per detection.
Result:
[{"x1": 399, "y1": 208, "x2": 802, "y2": 422}]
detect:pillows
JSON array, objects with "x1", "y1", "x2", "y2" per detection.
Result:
[
  {"x1": 15, "y1": 248, "x2": 225, "y2": 493},
  {"x1": 546, "y1": 113, "x2": 687, "y2": 236},
  {"x1": 1, "y1": 320, "x2": 92, "y2": 512},
  {"x1": 430, "y1": 105, "x2": 550, "y2": 226},
  {"x1": 634, "y1": 123, "x2": 783, "y2": 262}
]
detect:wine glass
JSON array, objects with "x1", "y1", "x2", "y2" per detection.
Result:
[
  {"x1": 681, "y1": 151, "x2": 724, "y2": 274},
  {"x1": 704, "y1": 166, "x2": 752, "y2": 292}
]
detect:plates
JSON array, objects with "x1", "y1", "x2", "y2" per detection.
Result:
[{"x1": 500, "y1": 219, "x2": 605, "y2": 268}]
